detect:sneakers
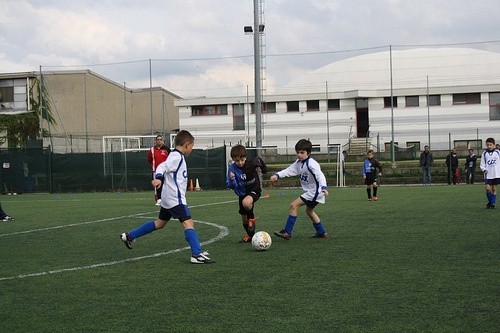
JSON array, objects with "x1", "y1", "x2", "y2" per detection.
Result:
[
  {"x1": 312, "y1": 232, "x2": 328, "y2": 238},
  {"x1": 121, "y1": 232, "x2": 135, "y2": 248},
  {"x1": 242, "y1": 234, "x2": 252, "y2": 242},
  {"x1": 190, "y1": 250, "x2": 215, "y2": 264},
  {"x1": 248, "y1": 216, "x2": 256, "y2": 227},
  {"x1": 274, "y1": 229, "x2": 291, "y2": 240}
]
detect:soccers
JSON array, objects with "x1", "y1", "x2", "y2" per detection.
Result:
[{"x1": 251, "y1": 230, "x2": 272, "y2": 251}]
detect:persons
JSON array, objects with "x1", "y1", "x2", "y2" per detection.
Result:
[
  {"x1": 120, "y1": 130, "x2": 215, "y2": 264},
  {"x1": 362, "y1": 150, "x2": 383, "y2": 201},
  {"x1": 480, "y1": 138, "x2": 500, "y2": 209},
  {"x1": 147, "y1": 135, "x2": 171, "y2": 206},
  {"x1": 270, "y1": 139, "x2": 328, "y2": 240},
  {"x1": 496, "y1": 144, "x2": 500, "y2": 150},
  {"x1": 0, "y1": 203, "x2": 14, "y2": 222},
  {"x1": 465, "y1": 149, "x2": 477, "y2": 184},
  {"x1": 420, "y1": 146, "x2": 432, "y2": 187},
  {"x1": 226, "y1": 144, "x2": 267, "y2": 243},
  {"x1": 446, "y1": 148, "x2": 459, "y2": 186}
]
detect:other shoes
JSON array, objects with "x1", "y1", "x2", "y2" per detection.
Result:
[
  {"x1": 373, "y1": 195, "x2": 378, "y2": 200},
  {"x1": 0, "y1": 216, "x2": 14, "y2": 221},
  {"x1": 368, "y1": 197, "x2": 372, "y2": 200},
  {"x1": 491, "y1": 203, "x2": 496, "y2": 209},
  {"x1": 155, "y1": 199, "x2": 162, "y2": 206},
  {"x1": 486, "y1": 201, "x2": 491, "y2": 208}
]
